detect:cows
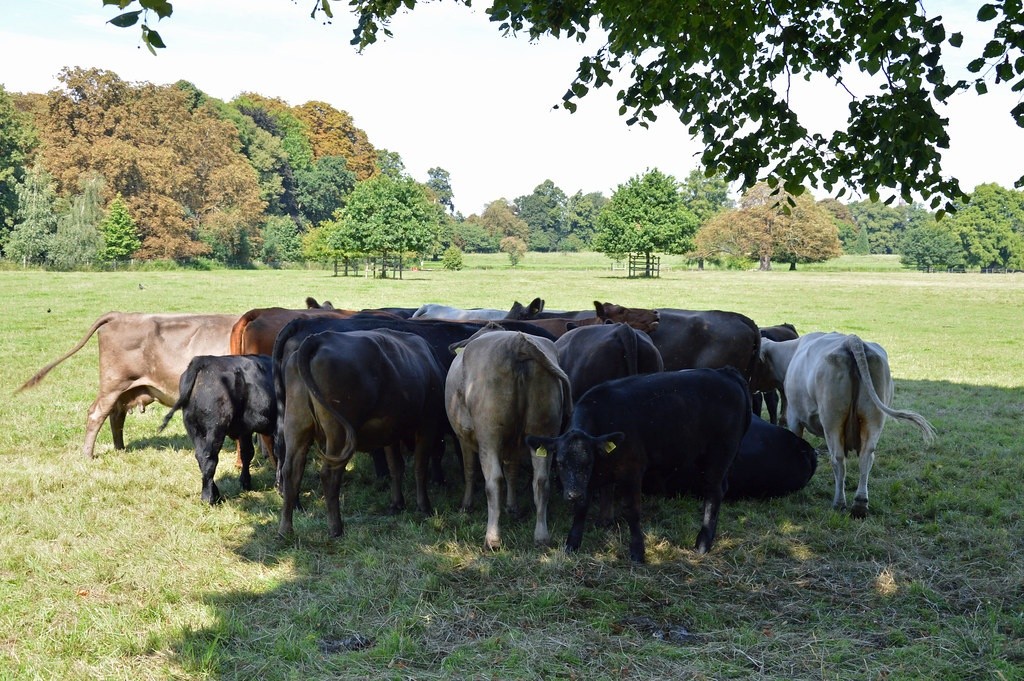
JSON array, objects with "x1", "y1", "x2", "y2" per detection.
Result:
[{"x1": 13, "y1": 296, "x2": 936, "y2": 565}]
